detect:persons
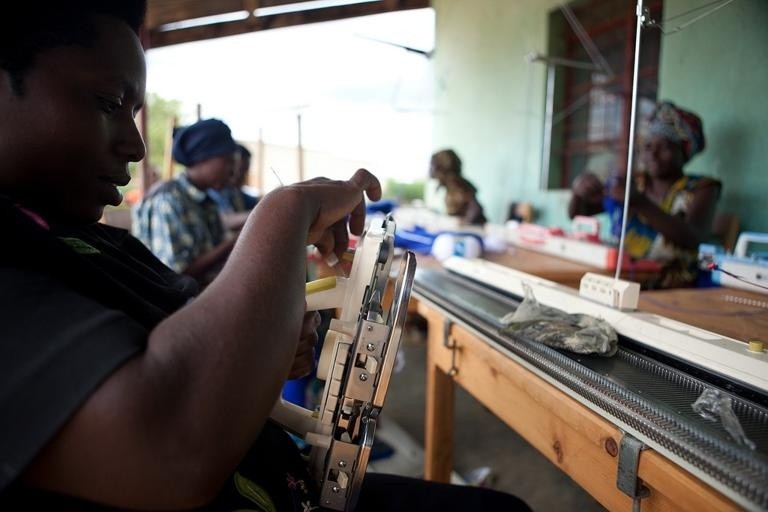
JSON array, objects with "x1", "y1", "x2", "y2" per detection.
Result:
[
  {"x1": 0, "y1": 0, "x2": 536, "y2": 507},
  {"x1": 430, "y1": 148, "x2": 487, "y2": 228},
  {"x1": 206, "y1": 150, "x2": 250, "y2": 241},
  {"x1": 238, "y1": 143, "x2": 261, "y2": 211},
  {"x1": 566, "y1": 100, "x2": 723, "y2": 255},
  {"x1": 130, "y1": 116, "x2": 240, "y2": 289}
]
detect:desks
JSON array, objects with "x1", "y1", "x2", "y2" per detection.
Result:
[{"x1": 380, "y1": 232, "x2": 768, "y2": 512}]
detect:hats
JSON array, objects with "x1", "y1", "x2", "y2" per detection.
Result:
[
  {"x1": 640, "y1": 100, "x2": 707, "y2": 162},
  {"x1": 172, "y1": 118, "x2": 242, "y2": 167}
]
